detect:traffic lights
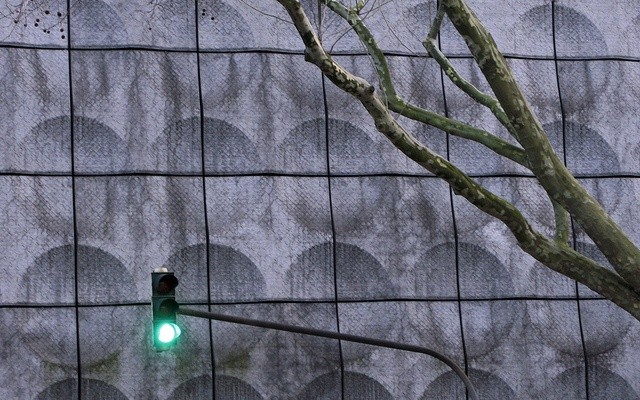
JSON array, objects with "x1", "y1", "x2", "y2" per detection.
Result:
[{"x1": 151, "y1": 272, "x2": 181, "y2": 353}]
